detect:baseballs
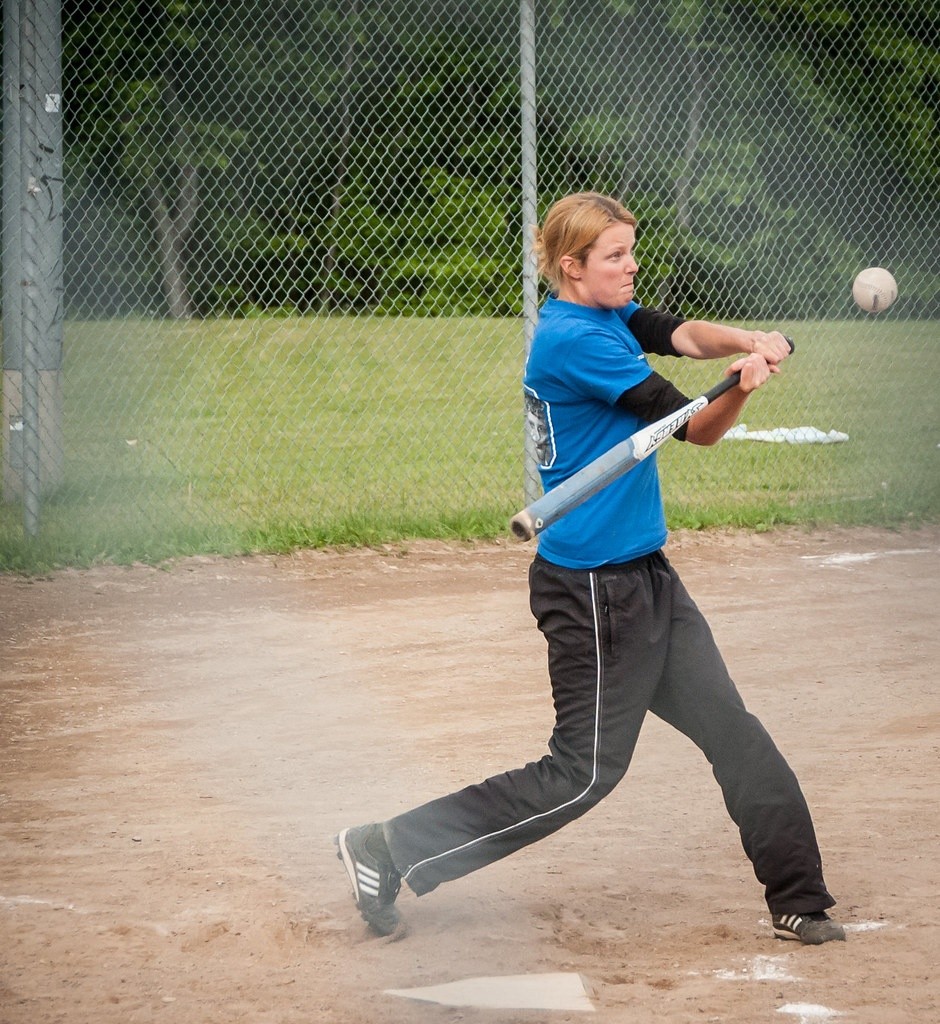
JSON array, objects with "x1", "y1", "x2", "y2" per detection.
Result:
[{"x1": 851, "y1": 266, "x2": 899, "y2": 312}]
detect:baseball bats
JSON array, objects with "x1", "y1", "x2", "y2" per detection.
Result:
[{"x1": 507, "y1": 333, "x2": 796, "y2": 543}]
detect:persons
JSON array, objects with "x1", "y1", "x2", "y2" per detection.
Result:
[{"x1": 330, "y1": 188, "x2": 850, "y2": 948}]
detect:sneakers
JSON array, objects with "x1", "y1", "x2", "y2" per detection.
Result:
[
  {"x1": 334, "y1": 823, "x2": 401, "y2": 936},
  {"x1": 772, "y1": 911, "x2": 846, "y2": 945}
]
